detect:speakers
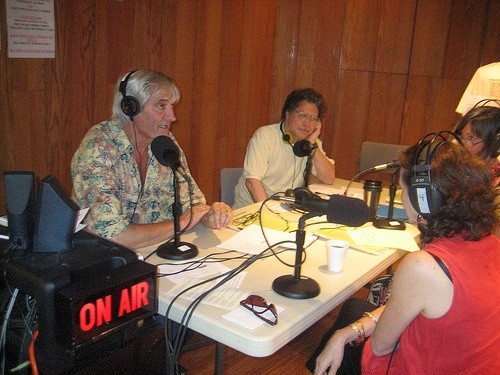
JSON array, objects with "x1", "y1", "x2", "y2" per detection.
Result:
[
  {"x1": 32, "y1": 175, "x2": 79, "y2": 252},
  {"x1": 3, "y1": 171, "x2": 35, "y2": 250}
]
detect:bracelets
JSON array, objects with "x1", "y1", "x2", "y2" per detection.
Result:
[
  {"x1": 349, "y1": 320, "x2": 365, "y2": 347},
  {"x1": 313, "y1": 143, "x2": 318, "y2": 150},
  {"x1": 363, "y1": 312, "x2": 378, "y2": 325}
]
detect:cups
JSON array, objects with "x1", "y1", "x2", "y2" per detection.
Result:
[{"x1": 325, "y1": 240, "x2": 350, "y2": 274}]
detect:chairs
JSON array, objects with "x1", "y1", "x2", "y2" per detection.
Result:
[
  {"x1": 356, "y1": 142, "x2": 408, "y2": 174},
  {"x1": 221, "y1": 168, "x2": 243, "y2": 207}
]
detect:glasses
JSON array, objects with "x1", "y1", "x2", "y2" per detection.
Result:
[
  {"x1": 240, "y1": 294, "x2": 278, "y2": 326},
  {"x1": 460, "y1": 138, "x2": 486, "y2": 148},
  {"x1": 292, "y1": 107, "x2": 319, "y2": 125}
]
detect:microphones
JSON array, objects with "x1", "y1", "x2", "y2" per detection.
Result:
[
  {"x1": 293, "y1": 140, "x2": 318, "y2": 157},
  {"x1": 374, "y1": 161, "x2": 401, "y2": 170},
  {"x1": 151, "y1": 135, "x2": 192, "y2": 184},
  {"x1": 271, "y1": 194, "x2": 369, "y2": 227}
]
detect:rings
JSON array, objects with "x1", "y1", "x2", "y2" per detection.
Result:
[{"x1": 226, "y1": 208, "x2": 232, "y2": 211}]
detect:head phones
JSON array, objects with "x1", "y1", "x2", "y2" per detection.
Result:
[
  {"x1": 471, "y1": 100, "x2": 500, "y2": 156},
  {"x1": 280, "y1": 120, "x2": 294, "y2": 144},
  {"x1": 408, "y1": 131, "x2": 463, "y2": 219},
  {"x1": 118, "y1": 69, "x2": 140, "y2": 117},
  {"x1": 286, "y1": 186, "x2": 314, "y2": 208}
]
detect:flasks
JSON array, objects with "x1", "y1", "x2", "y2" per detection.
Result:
[{"x1": 363, "y1": 180, "x2": 382, "y2": 221}]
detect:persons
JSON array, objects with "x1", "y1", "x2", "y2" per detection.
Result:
[
  {"x1": 233, "y1": 86, "x2": 336, "y2": 210},
  {"x1": 304, "y1": 139, "x2": 500, "y2": 375},
  {"x1": 454, "y1": 106, "x2": 500, "y2": 189},
  {"x1": 71, "y1": 68, "x2": 235, "y2": 346}
]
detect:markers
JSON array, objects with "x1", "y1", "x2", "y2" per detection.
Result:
[{"x1": 272, "y1": 195, "x2": 295, "y2": 202}]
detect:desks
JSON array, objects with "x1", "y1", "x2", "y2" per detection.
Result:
[{"x1": 134, "y1": 179, "x2": 422, "y2": 375}]
